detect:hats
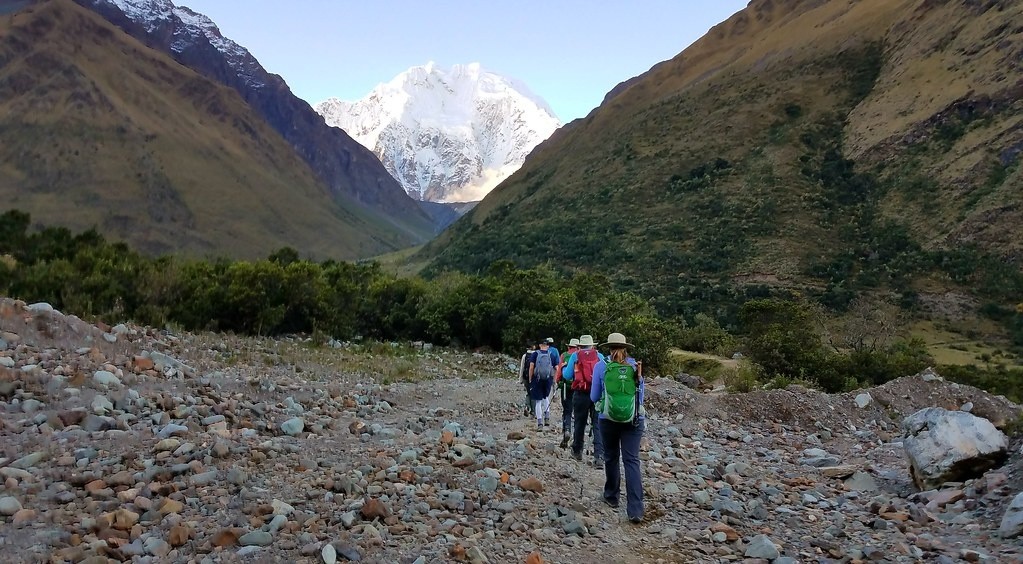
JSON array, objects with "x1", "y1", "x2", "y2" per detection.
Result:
[
  {"x1": 574, "y1": 335, "x2": 598, "y2": 345},
  {"x1": 539, "y1": 340, "x2": 550, "y2": 345},
  {"x1": 598, "y1": 333, "x2": 635, "y2": 348},
  {"x1": 565, "y1": 339, "x2": 579, "y2": 347},
  {"x1": 546, "y1": 338, "x2": 554, "y2": 342}
]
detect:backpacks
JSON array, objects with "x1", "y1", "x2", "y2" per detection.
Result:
[
  {"x1": 558, "y1": 352, "x2": 573, "y2": 388},
  {"x1": 601, "y1": 357, "x2": 634, "y2": 422},
  {"x1": 534, "y1": 350, "x2": 554, "y2": 381},
  {"x1": 571, "y1": 349, "x2": 599, "y2": 390},
  {"x1": 523, "y1": 352, "x2": 534, "y2": 380}
]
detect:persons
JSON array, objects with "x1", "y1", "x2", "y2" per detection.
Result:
[
  {"x1": 555, "y1": 335, "x2": 605, "y2": 469},
  {"x1": 529, "y1": 340, "x2": 558, "y2": 432},
  {"x1": 519, "y1": 338, "x2": 560, "y2": 419},
  {"x1": 590, "y1": 332, "x2": 645, "y2": 522}
]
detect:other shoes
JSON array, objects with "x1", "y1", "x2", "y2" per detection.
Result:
[
  {"x1": 570, "y1": 448, "x2": 583, "y2": 460},
  {"x1": 524, "y1": 407, "x2": 529, "y2": 417},
  {"x1": 545, "y1": 419, "x2": 550, "y2": 426},
  {"x1": 560, "y1": 431, "x2": 570, "y2": 447},
  {"x1": 630, "y1": 516, "x2": 644, "y2": 522},
  {"x1": 593, "y1": 458, "x2": 604, "y2": 468},
  {"x1": 602, "y1": 494, "x2": 619, "y2": 507},
  {"x1": 537, "y1": 425, "x2": 543, "y2": 431}
]
